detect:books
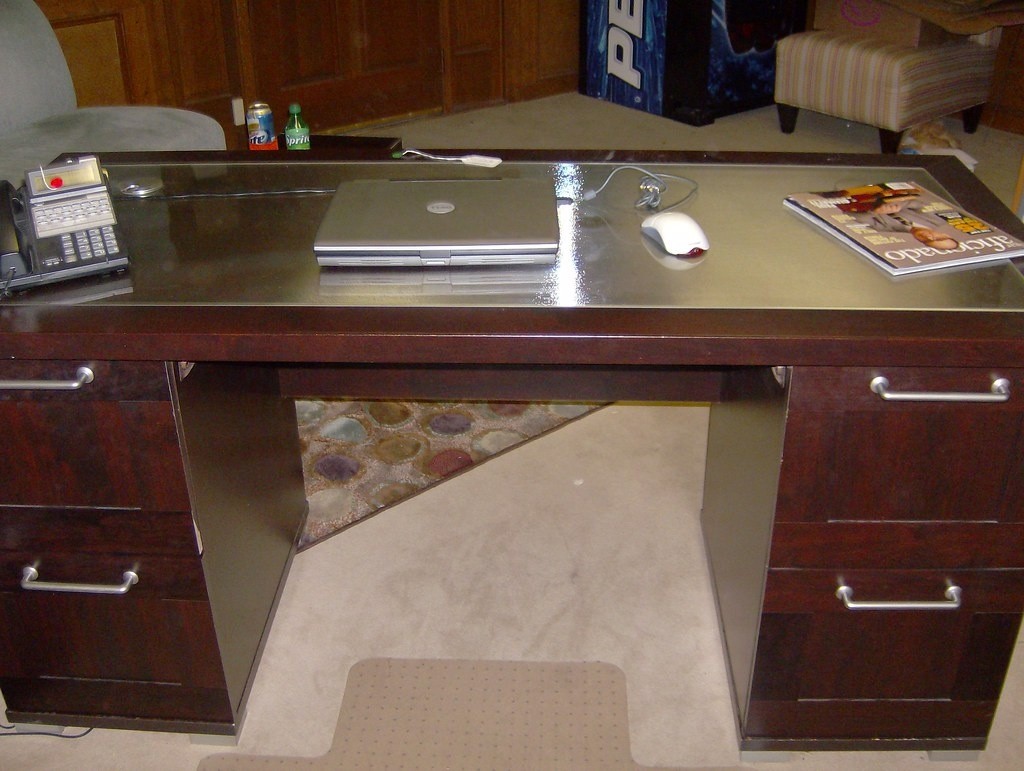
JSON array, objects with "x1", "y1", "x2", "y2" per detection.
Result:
[{"x1": 782, "y1": 180, "x2": 1024, "y2": 276}]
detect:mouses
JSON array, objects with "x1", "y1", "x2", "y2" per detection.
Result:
[{"x1": 641, "y1": 213, "x2": 710, "y2": 257}]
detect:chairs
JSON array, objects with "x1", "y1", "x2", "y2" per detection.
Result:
[
  {"x1": 774, "y1": 0, "x2": 1003, "y2": 155},
  {"x1": 0, "y1": 0, "x2": 228, "y2": 192}
]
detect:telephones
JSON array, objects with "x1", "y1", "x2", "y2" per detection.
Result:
[{"x1": 1, "y1": 157, "x2": 132, "y2": 299}]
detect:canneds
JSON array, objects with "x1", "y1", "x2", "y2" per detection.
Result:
[{"x1": 246, "y1": 103, "x2": 278, "y2": 150}]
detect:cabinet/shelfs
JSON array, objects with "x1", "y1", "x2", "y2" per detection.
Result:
[{"x1": 0, "y1": 149, "x2": 1024, "y2": 752}]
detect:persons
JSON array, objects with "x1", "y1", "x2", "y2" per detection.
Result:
[{"x1": 846, "y1": 199, "x2": 959, "y2": 251}]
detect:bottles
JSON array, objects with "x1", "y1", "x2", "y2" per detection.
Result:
[{"x1": 285, "y1": 103, "x2": 310, "y2": 151}]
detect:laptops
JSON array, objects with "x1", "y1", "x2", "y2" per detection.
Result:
[{"x1": 313, "y1": 176, "x2": 560, "y2": 266}]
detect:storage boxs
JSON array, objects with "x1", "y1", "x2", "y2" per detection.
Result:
[{"x1": 813, "y1": 0, "x2": 969, "y2": 49}]
